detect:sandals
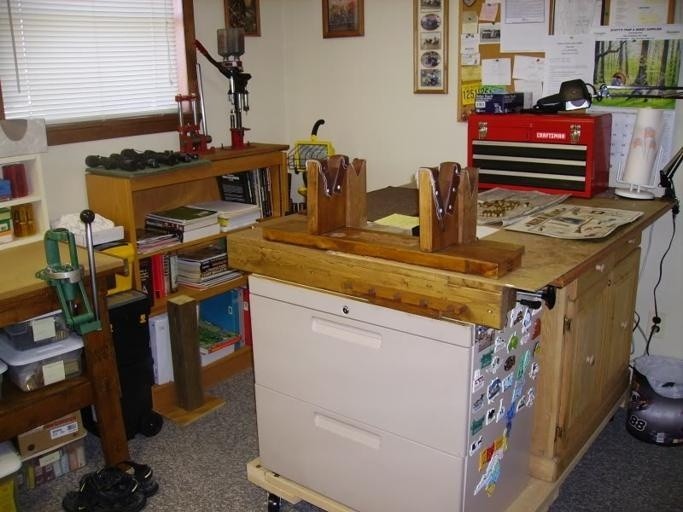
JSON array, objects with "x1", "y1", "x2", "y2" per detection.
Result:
[
  {"x1": 80, "y1": 461, "x2": 159, "y2": 497},
  {"x1": 62, "y1": 473, "x2": 147, "y2": 511}
]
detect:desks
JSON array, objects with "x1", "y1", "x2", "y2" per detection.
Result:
[{"x1": 1, "y1": 238, "x2": 132, "y2": 475}]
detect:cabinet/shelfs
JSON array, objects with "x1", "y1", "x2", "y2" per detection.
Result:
[
  {"x1": 86, "y1": 141, "x2": 289, "y2": 411},
  {"x1": 226, "y1": 169, "x2": 676, "y2": 512},
  {"x1": 2, "y1": 154, "x2": 51, "y2": 251}
]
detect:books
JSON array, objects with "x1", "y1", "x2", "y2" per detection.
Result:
[{"x1": 135, "y1": 166, "x2": 274, "y2": 368}]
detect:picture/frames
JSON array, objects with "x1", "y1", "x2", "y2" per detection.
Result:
[
  {"x1": 224, "y1": 0, "x2": 262, "y2": 38},
  {"x1": 413, "y1": 0, "x2": 449, "y2": 96},
  {"x1": 322, "y1": 0, "x2": 363, "y2": 39}
]
